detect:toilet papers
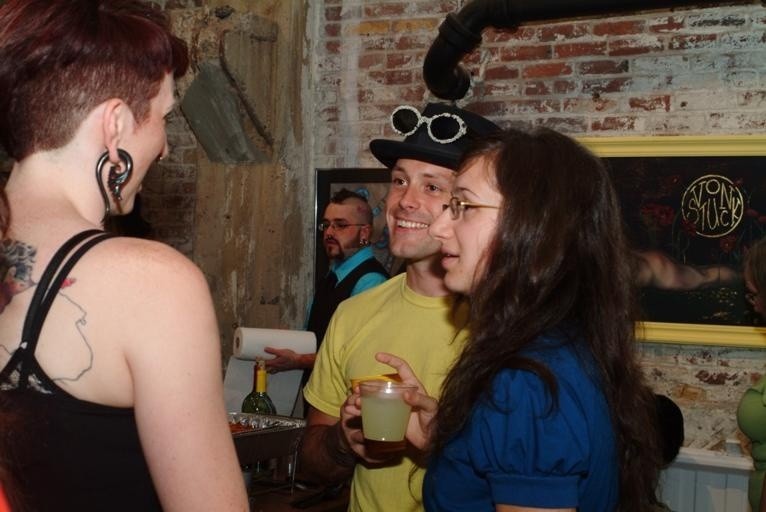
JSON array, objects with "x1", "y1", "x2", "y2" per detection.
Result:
[{"x1": 223, "y1": 325, "x2": 318, "y2": 419}]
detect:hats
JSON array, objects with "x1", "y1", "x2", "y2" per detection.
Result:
[{"x1": 368, "y1": 102, "x2": 506, "y2": 173}]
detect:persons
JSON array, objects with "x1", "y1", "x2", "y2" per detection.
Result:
[
  {"x1": 256, "y1": 188, "x2": 391, "y2": 374},
  {"x1": 345, "y1": 126, "x2": 656, "y2": 512},
  {"x1": 297, "y1": 102, "x2": 502, "y2": 512},
  {"x1": 0, "y1": 0, "x2": 251, "y2": 511}
]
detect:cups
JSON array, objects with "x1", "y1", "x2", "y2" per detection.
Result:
[{"x1": 359, "y1": 380, "x2": 418, "y2": 444}]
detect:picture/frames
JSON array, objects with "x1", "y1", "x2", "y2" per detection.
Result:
[
  {"x1": 570, "y1": 136, "x2": 766, "y2": 350},
  {"x1": 313, "y1": 166, "x2": 407, "y2": 297}
]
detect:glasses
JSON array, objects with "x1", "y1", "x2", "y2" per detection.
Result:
[
  {"x1": 389, "y1": 103, "x2": 483, "y2": 146},
  {"x1": 441, "y1": 195, "x2": 500, "y2": 220},
  {"x1": 318, "y1": 223, "x2": 365, "y2": 232}
]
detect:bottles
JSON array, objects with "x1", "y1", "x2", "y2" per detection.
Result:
[{"x1": 240, "y1": 362, "x2": 276, "y2": 416}]
